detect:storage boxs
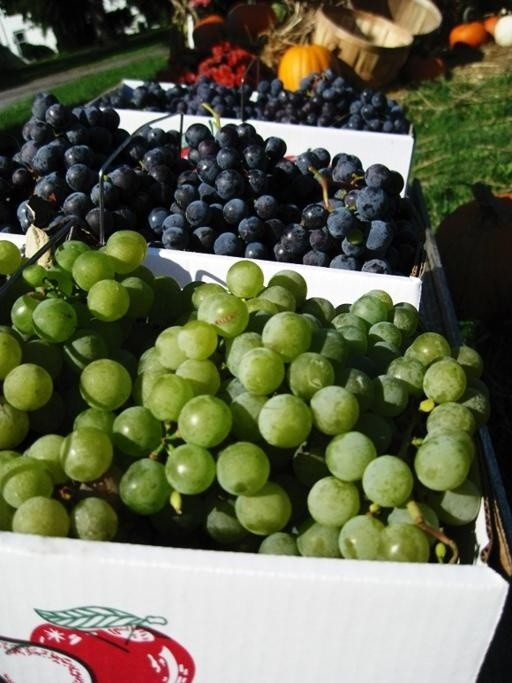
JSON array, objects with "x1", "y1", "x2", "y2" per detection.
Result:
[
  {"x1": 81, "y1": 76, "x2": 415, "y2": 200},
  {"x1": 0, "y1": 189, "x2": 425, "y2": 316},
  {"x1": 2, "y1": 432, "x2": 512, "y2": 681}
]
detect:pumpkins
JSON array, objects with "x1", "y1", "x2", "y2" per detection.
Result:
[
  {"x1": 278, "y1": 44, "x2": 338, "y2": 92},
  {"x1": 449, "y1": 15, "x2": 499, "y2": 48}
]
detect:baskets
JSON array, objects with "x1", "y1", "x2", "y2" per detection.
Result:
[
  {"x1": 346, "y1": 1, "x2": 445, "y2": 57},
  {"x1": 312, "y1": 5, "x2": 413, "y2": 94}
]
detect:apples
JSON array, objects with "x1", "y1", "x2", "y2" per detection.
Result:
[{"x1": 32, "y1": 620, "x2": 195, "y2": 683}]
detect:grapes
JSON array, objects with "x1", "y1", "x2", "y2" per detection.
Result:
[{"x1": 0, "y1": 69, "x2": 488, "y2": 563}]
